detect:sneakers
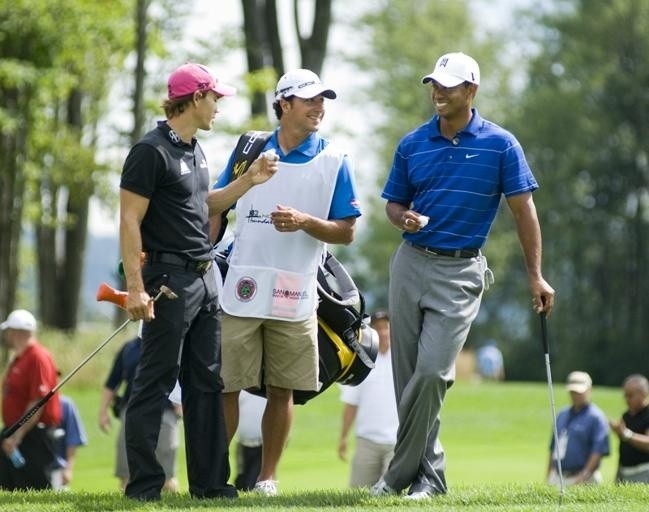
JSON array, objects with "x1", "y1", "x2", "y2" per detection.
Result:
[
  {"x1": 249, "y1": 477, "x2": 282, "y2": 498},
  {"x1": 401, "y1": 487, "x2": 449, "y2": 503},
  {"x1": 367, "y1": 473, "x2": 403, "y2": 500}
]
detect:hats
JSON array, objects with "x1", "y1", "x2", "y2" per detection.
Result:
[
  {"x1": 271, "y1": 68, "x2": 339, "y2": 104},
  {"x1": 418, "y1": 49, "x2": 483, "y2": 92},
  {"x1": 371, "y1": 311, "x2": 390, "y2": 323},
  {"x1": 165, "y1": 61, "x2": 234, "y2": 101},
  {"x1": 0, "y1": 308, "x2": 38, "y2": 333},
  {"x1": 564, "y1": 370, "x2": 593, "y2": 396}
]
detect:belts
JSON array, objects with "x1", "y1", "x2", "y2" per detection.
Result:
[
  {"x1": 410, "y1": 241, "x2": 481, "y2": 260},
  {"x1": 145, "y1": 249, "x2": 214, "y2": 275}
]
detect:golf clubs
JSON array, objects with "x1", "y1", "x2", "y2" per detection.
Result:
[
  {"x1": 539, "y1": 294, "x2": 566, "y2": 507},
  {"x1": 5, "y1": 285, "x2": 177, "y2": 440}
]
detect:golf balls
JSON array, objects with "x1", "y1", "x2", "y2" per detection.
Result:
[{"x1": 418, "y1": 217, "x2": 429, "y2": 225}]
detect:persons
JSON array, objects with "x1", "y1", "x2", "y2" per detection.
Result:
[
  {"x1": 547, "y1": 369, "x2": 611, "y2": 492},
  {"x1": 28, "y1": 368, "x2": 85, "y2": 492},
  {"x1": 1, "y1": 310, "x2": 63, "y2": 490},
  {"x1": 209, "y1": 68, "x2": 361, "y2": 497},
  {"x1": 119, "y1": 58, "x2": 280, "y2": 499},
  {"x1": 99, "y1": 314, "x2": 183, "y2": 493},
  {"x1": 371, "y1": 50, "x2": 555, "y2": 501},
  {"x1": 336, "y1": 309, "x2": 400, "y2": 491},
  {"x1": 607, "y1": 374, "x2": 648, "y2": 486},
  {"x1": 233, "y1": 387, "x2": 267, "y2": 491}
]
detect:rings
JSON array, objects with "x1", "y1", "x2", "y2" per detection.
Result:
[
  {"x1": 405, "y1": 218, "x2": 410, "y2": 224},
  {"x1": 282, "y1": 222, "x2": 285, "y2": 228}
]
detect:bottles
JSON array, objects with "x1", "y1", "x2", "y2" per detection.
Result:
[
  {"x1": 96, "y1": 282, "x2": 128, "y2": 311},
  {"x1": 10, "y1": 445, "x2": 25, "y2": 468}
]
